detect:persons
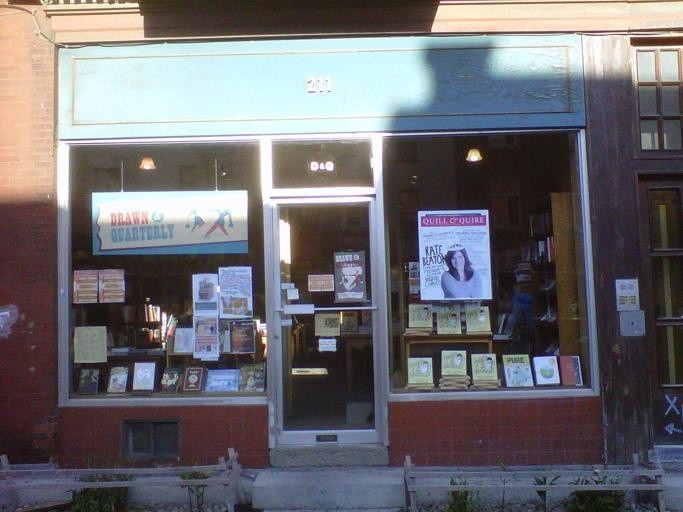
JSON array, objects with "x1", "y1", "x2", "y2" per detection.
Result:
[{"x1": 441, "y1": 244, "x2": 482, "y2": 298}]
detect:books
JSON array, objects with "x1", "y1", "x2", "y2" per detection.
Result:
[
  {"x1": 183, "y1": 368, "x2": 207, "y2": 392},
  {"x1": 502, "y1": 355, "x2": 534, "y2": 387},
  {"x1": 537, "y1": 306, "x2": 557, "y2": 321},
  {"x1": 162, "y1": 371, "x2": 182, "y2": 392},
  {"x1": 520, "y1": 236, "x2": 554, "y2": 264},
  {"x1": 529, "y1": 213, "x2": 552, "y2": 238},
  {"x1": 238, "y1": 363, "x2": 265, "y2": 392},
  {"x1": 107, "y1": 367, "x2": 128, "y2": 392},
  {"x1": 436, "y1": 304, "x2": 462, "y2": 334},
  {"x1": 406, "y1": 358, "x2": 434, "y2": 389},
  {"x1": 533, "y1": 356, "x2": 560, "y2": 386},
  {"x1": 439, "y1": 351, "x2": 470, "y2": 390},
  {"x1": 79, "y1": 369, "x2": 99, "y2": 394},
  {"x1": 403, "y1": 304, "x2": 432, "y2": 336},
  {"x1": 558, "y1": 356, "x2": 583, "y2": 386},
  {"x1": 538, "y1": 279, "x2": 556, "y2": 290},
  {"x1": 466, "y1": 307, "x2": 491, "y2": 331},
  {"x1": 133, "y1": 363, "x2": 155, "y2": 390},
  {"x1": 470, "y1": 354, "x2": 500, "y2": 389},
  {"x1": 111, "y1": 305, "x2": 177, "y2": 356}
]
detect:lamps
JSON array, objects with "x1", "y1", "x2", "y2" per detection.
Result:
[
  {"x1": 137, "y1": 147, "x2": 158, "y2": 171},
  {"x1": 463, "y1": 137, "x2": 482, "y2": 163}
]
